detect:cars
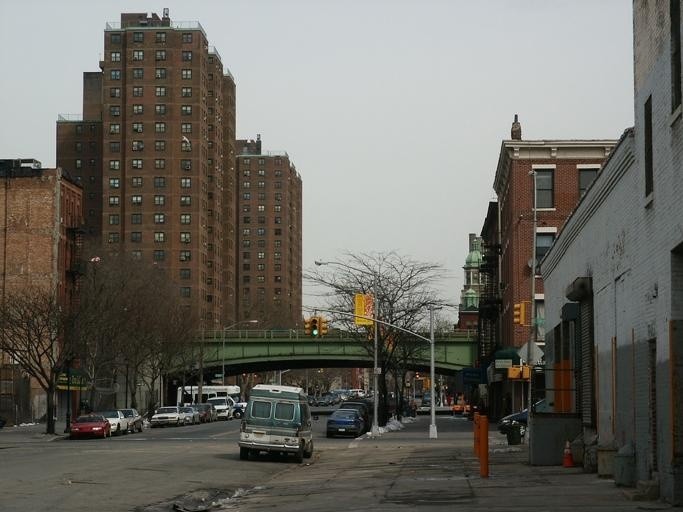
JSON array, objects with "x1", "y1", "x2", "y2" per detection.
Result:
[
  {"x1": 116, "y1": 408, "x2": 144, "y2": 434},
  {"x1": 497, "y1": 397, "x2": 546, "y2": 437},
  {"x1": 150, "y1": 405, "x2": 186, "y2": 427},
  {"x1": 326, "y1": 408, "x2": 365, "y2": 438},
  {"x1": 198, "y1": 396, "x2": 235, "y2": 423},
  {"x1": 70, "y1": 415, "x2": 111, "y2": 438},
  {"x1": 99, "y1": 410, "x2": 129, "y2": 436},
  {"x1": 183, "y1": 406, "x2": 201, "y2": 425}
]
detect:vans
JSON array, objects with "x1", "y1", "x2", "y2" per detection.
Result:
[{"x1": 238, "y1": 383, "x2": 319, "y2": 464}]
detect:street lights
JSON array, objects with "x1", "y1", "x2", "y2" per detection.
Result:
[
  {"x1": 314, "y1": 260, "x2": 382, "y2": 440},
  {"x1": 222, "y1": 319, "x2": 258, "y2": 386}
]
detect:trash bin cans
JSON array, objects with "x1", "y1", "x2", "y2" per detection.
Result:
[
  {"x1": 598, "y1": 449, "x2": 635, "y2": 486},
  {"x1": 506, "y1": 425, "x2": 521, "y2": 445}
]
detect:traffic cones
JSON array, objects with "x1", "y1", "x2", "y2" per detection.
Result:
[{"x1": 562, "y1": 438, "x2": 575, "y2": 468}]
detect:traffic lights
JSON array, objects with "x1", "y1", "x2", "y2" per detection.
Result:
[
  {"x1": 304, "y1": 318, "x2": 311, "y2": 335},
  {"x1": 311, "y1": 317, "x2": 319, "y2": 338},
  {"x1": 321, "y1": 317, "x2": 328, "y2": 336}
]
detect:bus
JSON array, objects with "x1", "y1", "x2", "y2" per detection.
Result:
[{"x1": 176, "y1": 383, "x2": 248, "y2": 419}]
